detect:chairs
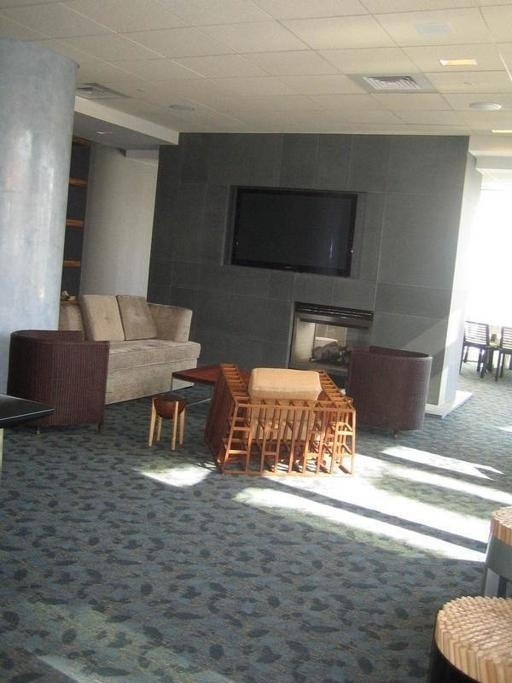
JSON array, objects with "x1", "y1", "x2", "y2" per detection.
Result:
[
  {"x1": 458, "y1": 320, "x2": 511, "y2": 383},
  {"x1": 344, "y1": 345, "x2": 433, "y2": 438},
  {"x1": 7, "y1": 329, "x2": 110, "y2": 435},
  {"x1": 203, "y1": 361, "x2": 357, "y2": 477}
]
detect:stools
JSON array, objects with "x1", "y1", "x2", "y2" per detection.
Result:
[{"x1": 146, "y1": 394, "x2": 188, "y2": 451}]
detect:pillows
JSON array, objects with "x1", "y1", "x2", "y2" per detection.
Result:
[{"x1": 79, "y1": 293, "x2": 158, "y2": 342}]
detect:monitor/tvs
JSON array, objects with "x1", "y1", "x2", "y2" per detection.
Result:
[{"x1": 230, "y1": 187, "x2": 358, "y2": 277}]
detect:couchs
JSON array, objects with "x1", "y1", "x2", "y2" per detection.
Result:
[{"x1": 60, "y1": 300, "x2": 203, "y2": 407}]
